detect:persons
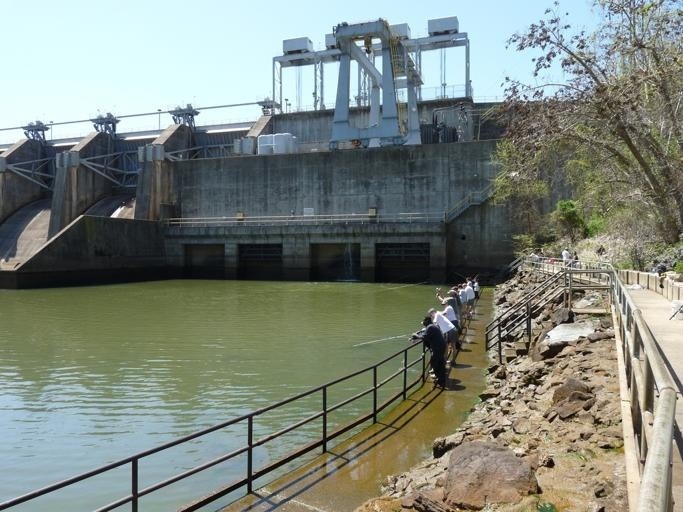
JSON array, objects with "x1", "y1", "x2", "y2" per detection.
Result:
[
  {"x1": 411, "y1": 277, "x2": 480, "y2": 387},
  {"x1": 528, "y1": 247, "x2": 578, "y2": 267}
]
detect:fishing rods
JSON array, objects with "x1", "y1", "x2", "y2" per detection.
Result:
[{"x1": 354, "y1": 334, "x2": 411, "y2": 348}]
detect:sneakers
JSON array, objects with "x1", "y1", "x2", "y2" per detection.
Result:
[
  {"x1": 446, "y1": 359, "x2": 456, "y2": 366},
  {"x1": 430, "y1": 373, "x2": 437, "y2": 378},
  {"x1": 429, "y1": 368, "x2": 435, "y2": 373}
]
